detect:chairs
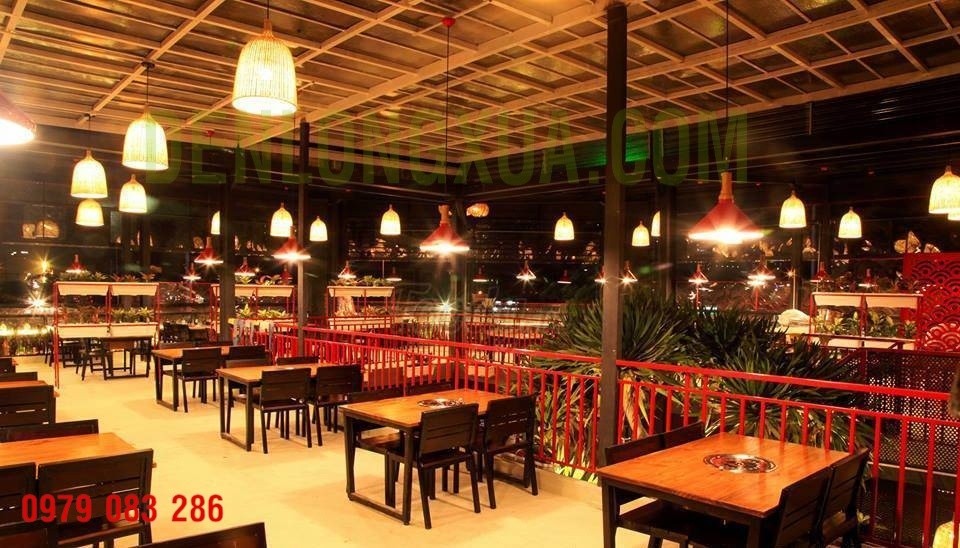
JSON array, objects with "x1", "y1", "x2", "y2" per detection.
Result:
[{"x1": 0, "y1": 324, "x2": 870, "y2": 548}]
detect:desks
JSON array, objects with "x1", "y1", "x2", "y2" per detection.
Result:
[
  {"x1": 0, "y1": 432, "x2": 153, "y2": 548},
  {"x1": 215, "y1": 363, "x2": 363, "y2": 451},
  {"x1": 0, "y1": 380, "x2": 59, "y2": 423},
  {"x1": 151, "y1": 346, "x2": 272, "y2": 412},
  {"x1": 338, "y1": 389, "x2": 530, "y2": 528},
  {"x1": 84, "y1": 336, "x2": 152, "y2": 381},
  {"x1": 597, "y1": 435, "x2": 850, "y2": 548}
]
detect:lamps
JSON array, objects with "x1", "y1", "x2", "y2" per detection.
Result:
[{"x1": 0, "y1": 0, "x2": 960, "y2": 310}]
